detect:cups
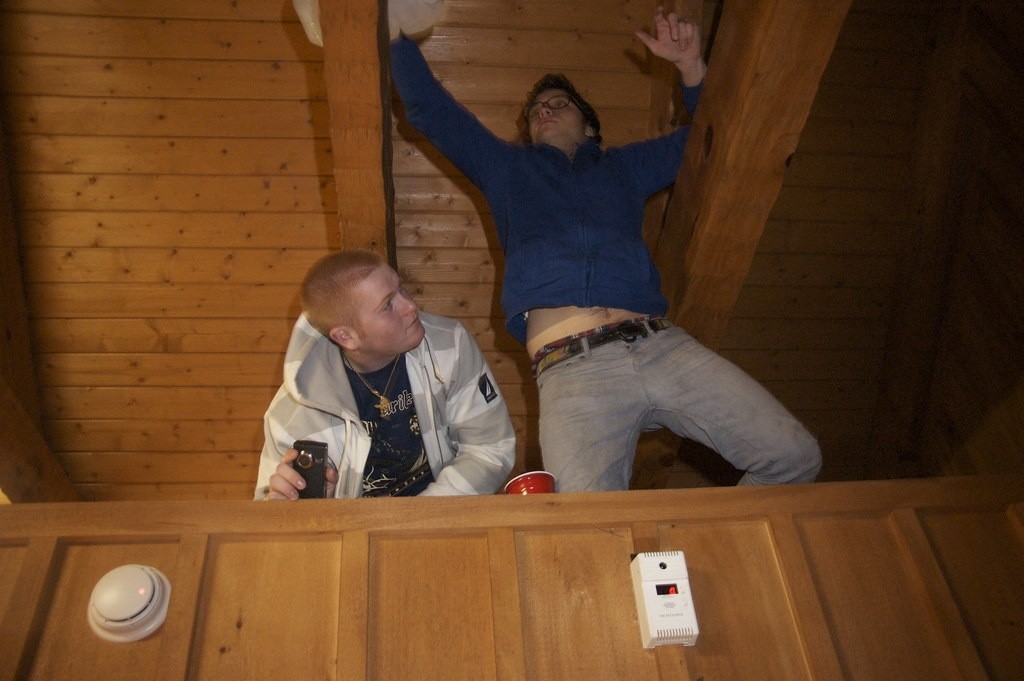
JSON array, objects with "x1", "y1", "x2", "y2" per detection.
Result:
[{"x1": 504, "y1": 471, "x2": 556, "y2": 495}]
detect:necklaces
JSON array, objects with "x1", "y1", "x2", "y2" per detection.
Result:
[{"x1": 342, "y1": 350, "x2": 401, "y2": 410}]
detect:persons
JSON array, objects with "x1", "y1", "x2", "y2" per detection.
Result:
[
  {"x1": 386, "y1": 6, "x2": 824, "y2": 496},
  {"x1": 251, "y1": 251, "x2": 514, "y2": 502}
]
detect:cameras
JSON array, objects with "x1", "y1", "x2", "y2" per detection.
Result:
[{"x1": 292, "y1": 440, "x2": 328, "y2": 499}]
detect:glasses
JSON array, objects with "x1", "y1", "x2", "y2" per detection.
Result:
[{"x1": 524, "y1": 94, "x2": 584, "y2": 117}]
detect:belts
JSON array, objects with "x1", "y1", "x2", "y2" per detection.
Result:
[{"x1": 537, "y1": 320, "x2": 671, "y2": 376}]
